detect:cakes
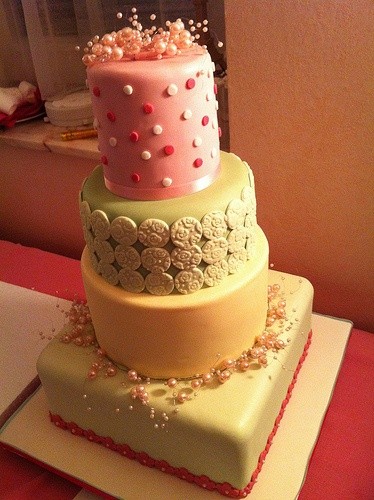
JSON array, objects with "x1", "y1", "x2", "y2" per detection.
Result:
[{"x1": 36, "y1": 38, "x2": 314, "y2": 498}]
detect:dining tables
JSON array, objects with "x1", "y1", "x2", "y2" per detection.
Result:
[{"x1": 0, "y1": 238, "x2": 374, "y2": 499}]
